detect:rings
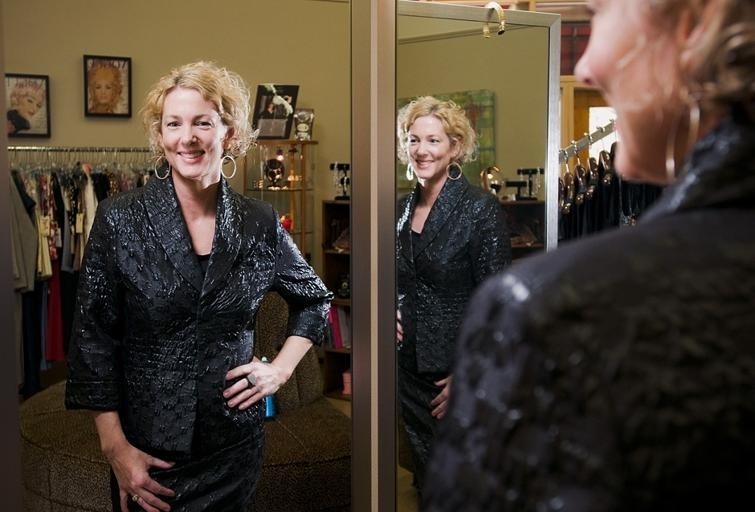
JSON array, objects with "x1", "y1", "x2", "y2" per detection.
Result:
[
  {"x1": 246, "y1": 374, "x2": 255, "y2": 390},
  {"x1": 131, "y1": 494, "x2": 139, "y2": 503}
]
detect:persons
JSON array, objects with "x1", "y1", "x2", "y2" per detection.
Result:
[
  {"x1": 87, "y1": 63, "x2": 122, "y2": 113},
  {"x1": 421, "y1": 0, "x2": 755, "y2": 512},
  {"x1": 259, "y1": 102, "x2": 274, "y2": 118},
  {"x1": 6, "y1": 82, "x2": 44, "y2": 134},
  {"x1": 395, "y1": 96, "x2": 513, "y2": 494},
  {"x1": 64, "y1": 61, "x2": 335, "y2": 512}
]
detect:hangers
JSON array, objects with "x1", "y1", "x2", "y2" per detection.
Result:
[{"x1": 558, "y1": 119, "x2": 620, "y2": 220}]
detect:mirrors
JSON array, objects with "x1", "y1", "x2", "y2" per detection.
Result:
[
  {"x1": 1, "y1": 2, "x2": 347, "y2": 511},
  {"x1": 395, "y1": 13, "x2": 551, "y2": 511}
]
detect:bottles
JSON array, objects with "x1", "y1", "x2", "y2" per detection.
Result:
[{"x1": 260, "y1": 356, "x2": 276, "y2": 419}]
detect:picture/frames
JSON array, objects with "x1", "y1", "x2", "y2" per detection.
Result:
[
  {"x1": 251, "y1": 85, "x2": 299, "y2": 140},
  {"x1": 6, "y1": 74, "x2": 52, "y2": 139},
  {"x1": 82, "y1": 55, "x2": 132, "y2": 118}
]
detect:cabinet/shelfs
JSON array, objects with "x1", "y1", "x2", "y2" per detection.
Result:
[
  {"x1": 322, "y1": 202, "x2": 352, "y2": 400},
  {"x1": 242, "y1": 139, "x2": 318, "y2": 264}
]
